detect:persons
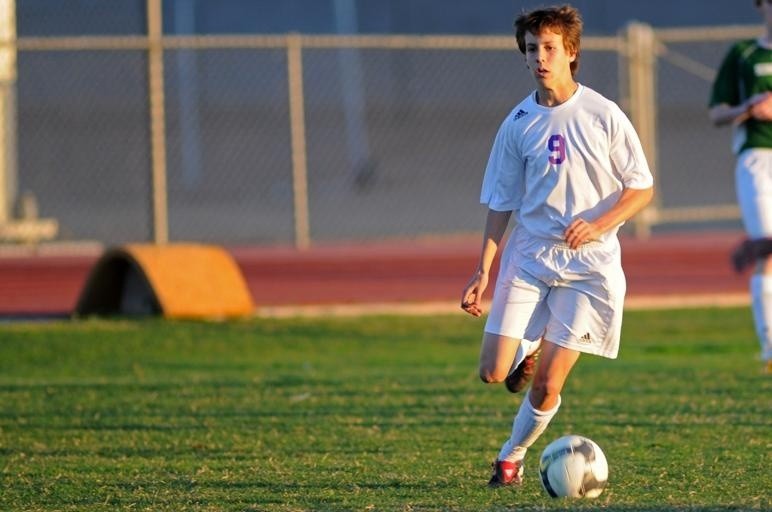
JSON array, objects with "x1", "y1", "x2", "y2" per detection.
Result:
[
  {"x1": 705, "y1": 0, "x2": 772, "y2": 363},
  {"x1": 460, "y1": 2, "x2": 654, "y2": 489},
  {"x1": 349, "y1": 0, "x2": 418, "y2": 188}
]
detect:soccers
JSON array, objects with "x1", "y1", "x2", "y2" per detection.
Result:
[{"x1": 539, "y1": 435, "x2": 609, "y2": 499}]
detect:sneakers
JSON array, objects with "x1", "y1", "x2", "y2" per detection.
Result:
[
  {"x1": 487, "y1": 458, "x2": 524, "y2": 490},
  {"x1": 505, "y1": 336, "x2": 541, "y2": 394}
]
